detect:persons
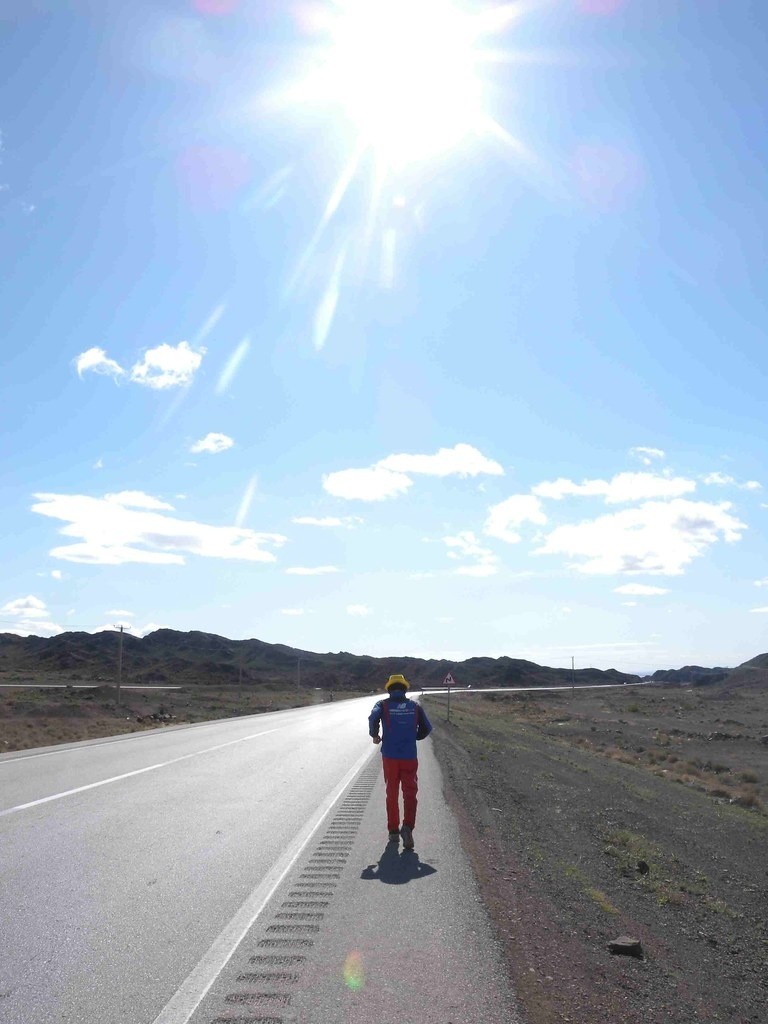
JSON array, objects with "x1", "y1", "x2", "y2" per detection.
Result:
[{"x1": 368, "y1": 675, "x2": 433, "y2": 848}]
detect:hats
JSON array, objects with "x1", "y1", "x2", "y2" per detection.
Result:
[{"x1": 386, "y1": 674, "x2": 409, "y2": 691}]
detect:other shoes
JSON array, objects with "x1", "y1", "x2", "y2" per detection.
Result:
[
  {"x1": 400, "y1": 825, "x2": 414, "y2": 849},
  {"x1": 389, "y1": 832, "x2": 399, "y2": 841}
]
